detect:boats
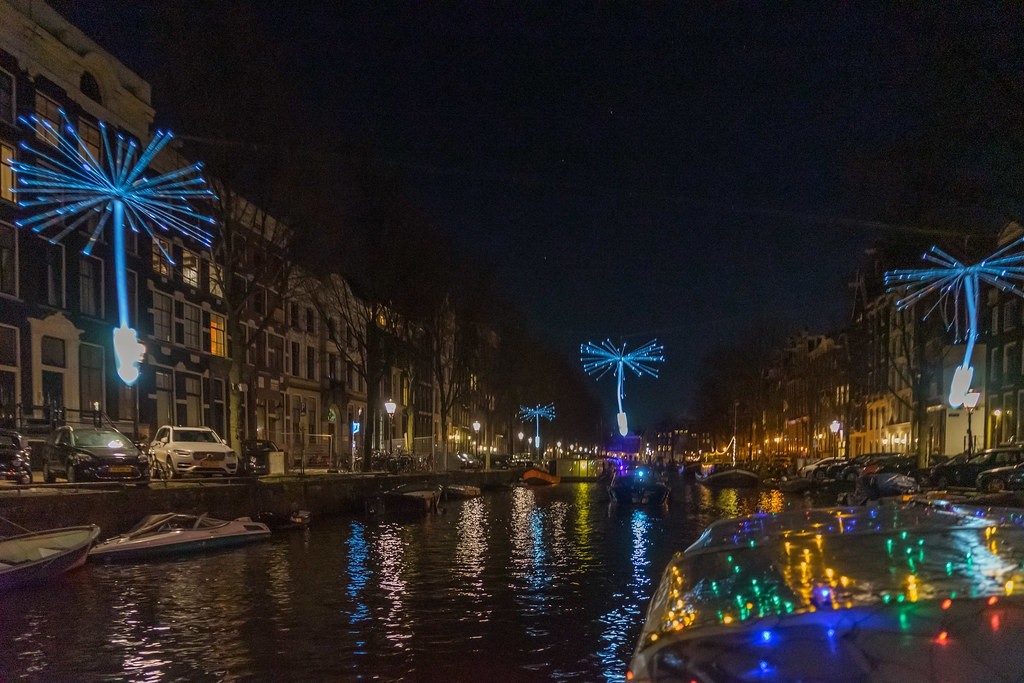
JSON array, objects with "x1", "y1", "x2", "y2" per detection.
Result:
[
  {"x1": 606, "y1": 464, "x2": 671, "y2": 504},
  {"x1": 88, "y1": 513, "x2": 272, "y2": 562},
  {"x1": 830, "y1": 420, "x2": 840, "y2": 460},
  {"x1": 522, "y1": 466, "x2": 561, "y2": 485},
  {"x1": 383, "y1": 481, "x2": 443, "y2": 517},
  {"x1": 447, "y1": 482, "x2": 482, "y2": 498},
  {"x1": 962, "y1": 389, "x2": 980, "y2": 458},
  {"x1": 0, "y1": 517, "x2": 101, "y2": 594},
  {"x1": 694, "y1": 462, "x2": 758, "y2": 488}
]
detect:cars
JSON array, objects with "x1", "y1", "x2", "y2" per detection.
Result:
[
  {"x1": 42, "y1": 425, "x2": 150, "y2": 486},
  {"x1": 240, "y1": 439, "x2": 284, "y2": 477},
  {"x1": 0, "y1": 429, "x2": 33, "y2": 485},
  {"x1": 770, "y1": 446, "x2": 1024, "y2": 497}
]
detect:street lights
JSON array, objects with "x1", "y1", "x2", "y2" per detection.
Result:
[
  {"x1": 130, "y1": 342, "x2": 147, "y2": 450},
  {"x1": 472, "y1": 420, "x2": 481, "y2": 458},
  {"x1": 385, "y1": 398, "x2": 396, "y2": 453},
  {"x1": 516, "y1": 431, "x2": 591, "y2": 465}
]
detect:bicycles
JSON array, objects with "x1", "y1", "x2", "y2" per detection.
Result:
[{"x1": 336, "y1": 448, "x2": 438, "y2": 474}]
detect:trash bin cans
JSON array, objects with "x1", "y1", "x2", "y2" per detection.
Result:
[{"x1": 268, "y1": 451, "x2": 285, "y2": 476}]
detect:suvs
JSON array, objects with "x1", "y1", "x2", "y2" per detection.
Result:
[{"x1": 148, "y1": 424, "x2": 239, "y2": 480}]
